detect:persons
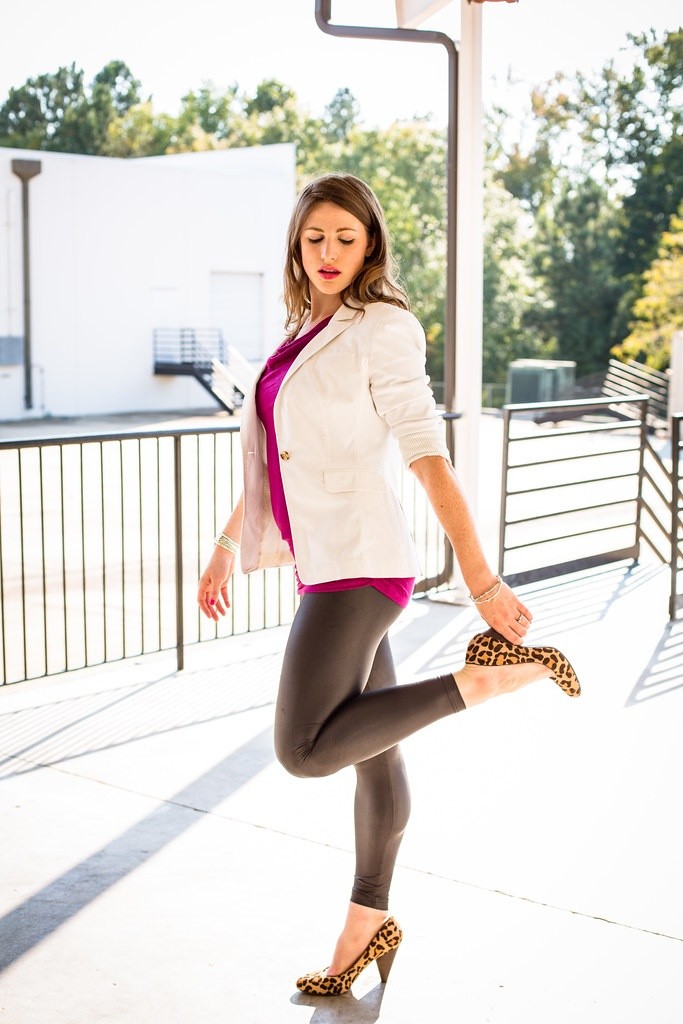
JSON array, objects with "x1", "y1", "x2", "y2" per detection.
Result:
[{"x1": 196, "y1": 174, "x2": 579, "y2": 994}]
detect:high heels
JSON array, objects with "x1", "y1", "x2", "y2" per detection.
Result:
[
  {"x1": 296, "y1": 915, "x2": 403, "y2": 997},
  {"x1": 465, "y1": 627, "x2": 581, "y2": 698}
]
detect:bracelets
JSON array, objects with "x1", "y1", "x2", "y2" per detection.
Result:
[
  {"x1": 467, "y1": 574, "x2": 503, "y2": 605},
  {"x1": 212, "y1": 534, "x2": 240, "y2": 556}
]
